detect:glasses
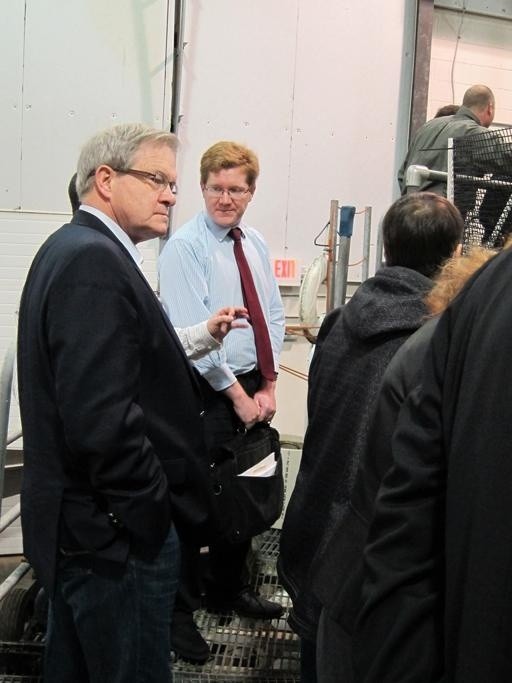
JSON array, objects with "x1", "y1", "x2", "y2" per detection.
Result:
[
  {"x1": 203, "y1": 185, "x2": 250, "y2": 197},
  {"x1": 113, "y1": 169, "x2": 178, "y2": 194}
]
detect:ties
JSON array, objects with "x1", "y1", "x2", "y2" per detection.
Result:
[{"x1": 227, "y1": 227, "x2": 276, "y2": 382}]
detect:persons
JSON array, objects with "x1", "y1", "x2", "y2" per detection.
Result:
[
  {"x1": 16, "y1": 120, "x2": 200, "y2": 682},
  {"x1": 312, "y1": 241, "x2": 500, "y2": 682},
  {"x1": 395, "y1": 82, "x2": 511, "y2": 235},
  {"x1": 154, "y1": 141, "x2": 285, "y2": 667},
  {"x1": 347, "y1": 240, "x2": 511, "y2": 681},
  {"x1": 174, "y1": 306, "x2": 251, "y2": 361},
  {"x1": 272, "y1": 189, "x2": 464, "y2": 682}
]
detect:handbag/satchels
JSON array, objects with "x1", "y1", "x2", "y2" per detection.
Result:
[{"x1": 206, "y1": 420, "x2": 283, "y2": 540}]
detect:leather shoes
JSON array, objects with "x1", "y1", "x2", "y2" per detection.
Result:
[
  {"x1": 207, "y1": 589, "x2": 282, "y2": 619},
  {"x1": 171, "y1": 612, "x2": 209, "y2": 664}
]
